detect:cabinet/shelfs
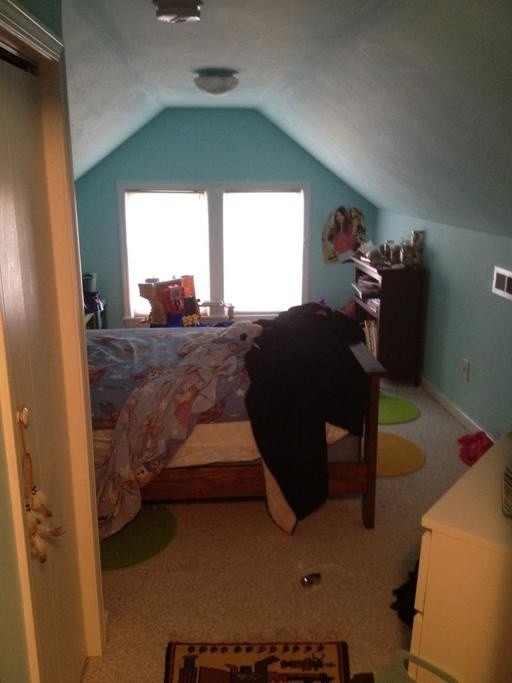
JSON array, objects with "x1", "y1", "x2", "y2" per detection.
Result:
[
  {"x1": 92, "y1": 298, "x2": 108, "y2": 330},
  {"x1": 407, "y1": 432, "x2": 512, "y2": 683},
  {"x1": 350, "y1": 255, "x2": 427, "y2": 389}
]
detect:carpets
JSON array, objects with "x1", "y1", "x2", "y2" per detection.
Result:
[
  {"x1": 163, "y1": 640, "x2": 352, "y2": 683},
  {"x1": 377, "y1": 392, "x2": 418, "y2": 425},
  {"x1": 378, "y1": 432, "x2": 424, "y2": 478}
]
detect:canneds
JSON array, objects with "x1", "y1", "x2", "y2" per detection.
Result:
[{"x1": 399, "y1": 231, "x2": 423, "y2": 266}]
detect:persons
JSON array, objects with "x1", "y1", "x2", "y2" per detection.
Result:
[{"x1": 330, "y1": 205, "x2": 358, "y2": 253}]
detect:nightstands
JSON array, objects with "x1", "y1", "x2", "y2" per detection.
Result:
[{"x1": 85, "y1": 313, "x2": 95, "y2": 329}]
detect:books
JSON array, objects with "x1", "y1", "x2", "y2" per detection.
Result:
[{"x1": 349, "y1": 273, "x2": 380, "y2": 359}]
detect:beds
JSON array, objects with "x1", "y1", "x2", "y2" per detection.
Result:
[{"x1": 84, "y1": 331, "x2": 387, "y2": 531}]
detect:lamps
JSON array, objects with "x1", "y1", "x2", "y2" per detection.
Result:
[{"x1": 191, "y1": 67, "x2": 241, "y2": 97}]
